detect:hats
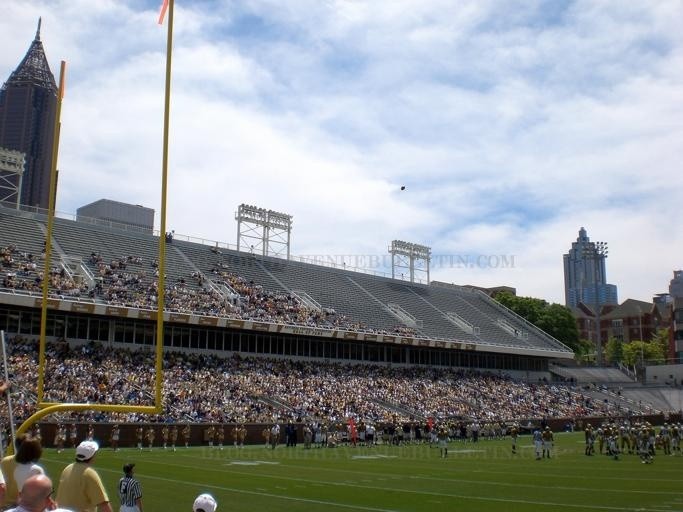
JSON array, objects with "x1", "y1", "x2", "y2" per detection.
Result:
[
  {"x1": 74, "y1": 440, "x2": 101, "y2": 463},
  {"x1": 122, "y1": 463, "x2": 136, "y2": 474}
]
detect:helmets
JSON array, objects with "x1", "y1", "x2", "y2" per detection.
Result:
[
  {"x1": 432, "y1": 424, "x2": 444, "y2": 430},
  {"x1": 584, "y1": 420, "x2": 681, "y2": 433},
  {"x1": 512, "y1": 425, "x2": 551, "y2": 431}
]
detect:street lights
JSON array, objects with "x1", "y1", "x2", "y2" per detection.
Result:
[
  {"x1": 582, "y1": 241, "x2": 609, "y2": 366},
  {"x1": 636, "y1": 304, "x2": 644, "y2": 368}
]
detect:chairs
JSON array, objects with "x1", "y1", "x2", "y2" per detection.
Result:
[{"x1": 0, "y1": 203, "x2": 574, "y2": 360}]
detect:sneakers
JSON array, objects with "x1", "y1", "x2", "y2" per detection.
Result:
[
  {"x1": 582, "y1": 447, "x2": 683, "y2": 465},
  {"x1": 509, "y1": 450, "x2": 552, "y2": 461}
]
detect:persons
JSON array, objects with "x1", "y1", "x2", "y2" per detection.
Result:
[
  {"x1": 354, "y1": 322, "x2": 413, "y2": 336},
  {"x1": 12, "y1": 438, "x2": 45, "y2": 505},
  {"x1": 117, "y1": 462, "x2": 142, "y2": 511},
  {"x1": 0, "y1": 242, "x2": 356, "y2": 333},
  {"x1": 0, "y1": 467, "x2": 8, "y2": 496},
  {"x1": 0, "y1": 333, "x2": 682, "y2": 464},
  {"x1": 1, "y1": 473, "x2": 71, "y2": 511},
  {"x1": 53, "y1": 440, "x2": 114, "y2": 511},
  {"x1": 0, "y1": 432, "x2": 34, "y2": 512}
]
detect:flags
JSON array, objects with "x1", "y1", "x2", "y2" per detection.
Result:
[
  {"x1": 56, "y1": 59, "x2": 66, "y2": 102},
  {"x1": 157, "y1": 0, "x2": 170, "y2": 26}
]
